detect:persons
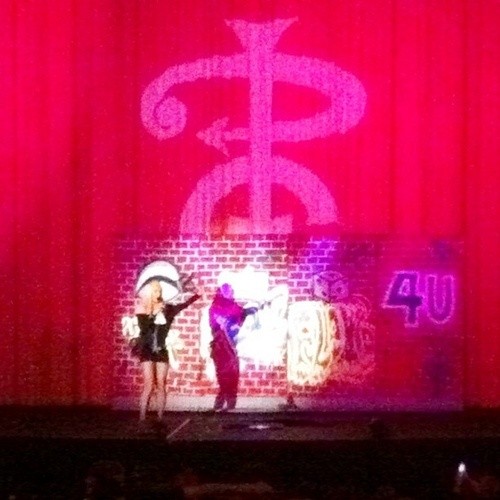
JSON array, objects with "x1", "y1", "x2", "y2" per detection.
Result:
[
  {"x1": 208, "y1": 283, "x2": 260, "y2": 414},
  {"x1": 131, "y1": 279, "x2": 204, "y2": 428}
]
